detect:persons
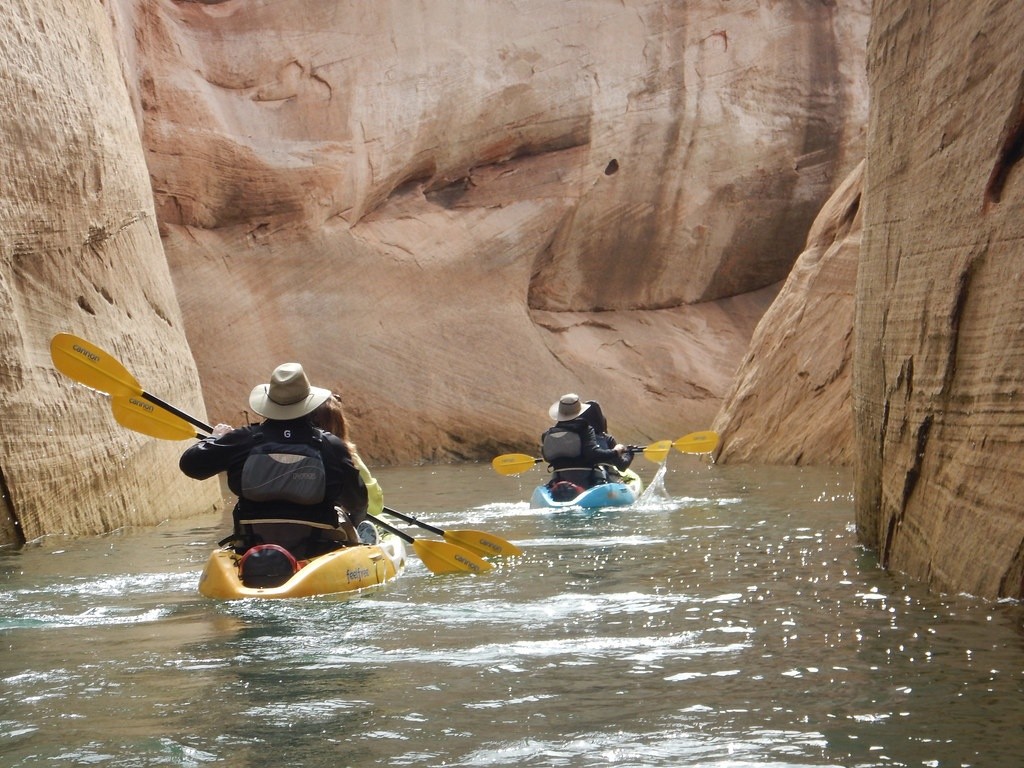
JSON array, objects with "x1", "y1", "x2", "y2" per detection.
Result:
[
  {"x1": 179, "y1": 363, "x2": 383, "y2": 558},
  {"x1": 542, "y1": 393, "x2": 634, "y2": 488}
]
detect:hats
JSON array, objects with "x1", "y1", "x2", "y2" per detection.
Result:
[
  {"x1": 249, "y1": 363, "x2": 331, "y2": 420},
  {"x1": 549, "y1": 393, "x2": 591, "y2": 421}
]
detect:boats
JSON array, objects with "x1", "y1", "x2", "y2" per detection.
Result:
[
  {"x1": 526, "y1": 467, "x2": 645, "y2": 512},
  {"x1": 195, "y1": 529, "x2": 406, "y2": 601}
]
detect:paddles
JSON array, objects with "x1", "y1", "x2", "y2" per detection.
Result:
[
  {"x1": 632, "y1": 429, "x2": 720, "y2": 456},
  {"x1": 47, "y1": 327, "x2": 499, "y2": 577},
  {"x1": 491, "y1": 438, "x2": 675, "y2": 478},
  {"x1": 107, "y1": 390, "x2": 528, "y2": 565}
]
